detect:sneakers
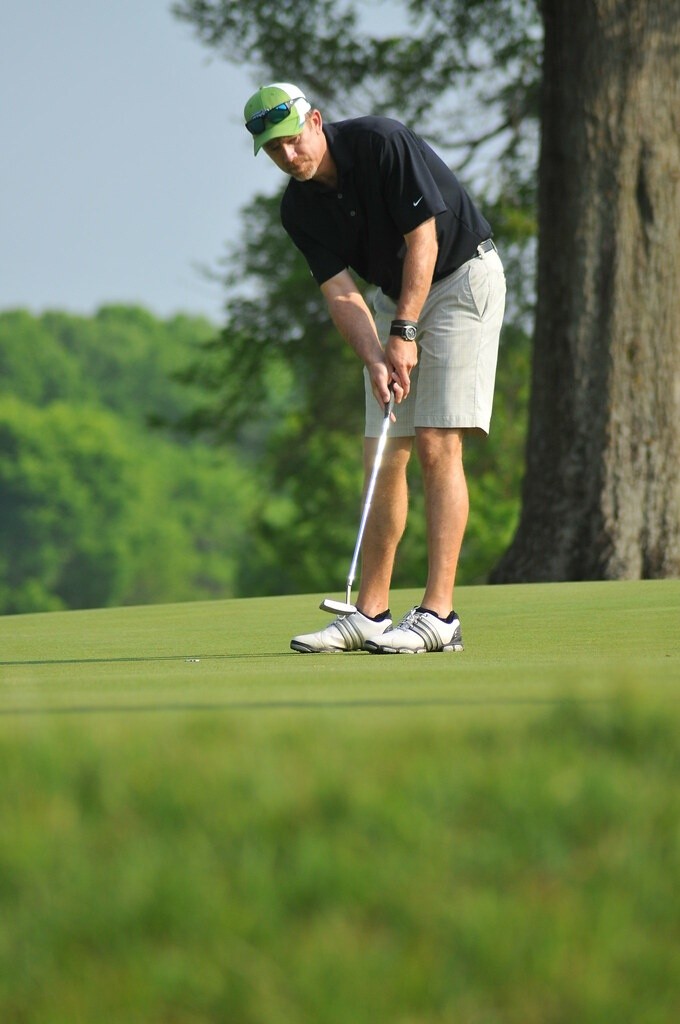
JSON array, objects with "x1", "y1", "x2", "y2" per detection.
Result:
[
  {"x1": 363, "y1": 604, "x2": 463, "y2": 653},
  {"x1": 289, "y1": 604, "x2": 393, "y2": 654}
]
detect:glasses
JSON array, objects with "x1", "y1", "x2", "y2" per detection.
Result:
[{"x1": 245, "y1": 96, "x2": 309, "y2": 134}]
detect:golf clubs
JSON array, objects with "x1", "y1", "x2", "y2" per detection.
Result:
[{"x1": 319, "y1": 368, "x2": 398, "y2": 616}]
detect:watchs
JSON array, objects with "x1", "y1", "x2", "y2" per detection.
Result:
[{"x1": 390, "y1": 326, "x2": 417, "y2": 341}]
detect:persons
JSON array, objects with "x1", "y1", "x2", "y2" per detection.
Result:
[{"x1": 244, "y1": 83, "x2": 507, "y2": 654}]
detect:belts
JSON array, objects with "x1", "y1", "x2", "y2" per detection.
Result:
[{"x1": 470, "y1": 239, "x2": 495, "y2": 258}]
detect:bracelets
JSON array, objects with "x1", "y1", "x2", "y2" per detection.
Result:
[{"x1": 391, "y1": 319, "x2": 419, "y2": 329}]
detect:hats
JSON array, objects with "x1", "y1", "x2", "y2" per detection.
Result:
[{"x1": 244, "y1": 83, "x2": 311, "y2": 156}]
